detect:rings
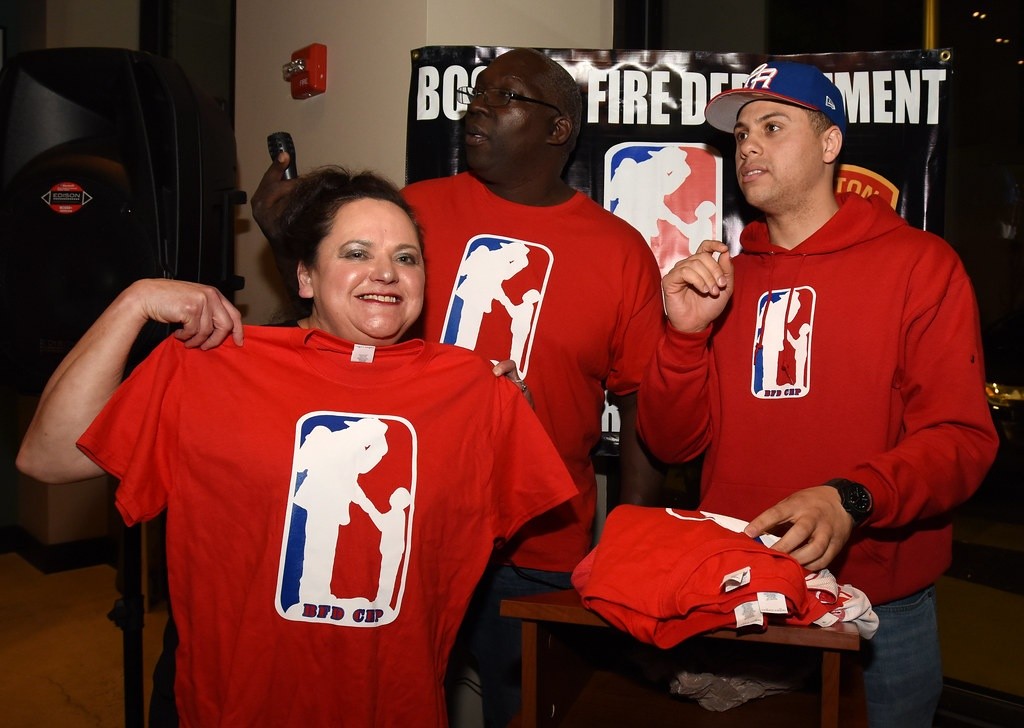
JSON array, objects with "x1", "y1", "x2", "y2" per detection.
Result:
[{"x1": 522, "y1": 384, "x2": 527, "y2": 392}]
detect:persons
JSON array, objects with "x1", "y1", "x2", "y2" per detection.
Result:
[
  {"x1": 251, "y1": 50, "x2": 664, "y2": 728},
  {"x1": 15, "y1": 163, "x2": 530, "y2": 728},
  {"x1": 635, "y1": 61, "x2": 999, "y2": 728}
]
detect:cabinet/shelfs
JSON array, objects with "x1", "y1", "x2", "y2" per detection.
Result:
[{"x1": 499, "y1": 588, "x2": 868, "y2": 728}]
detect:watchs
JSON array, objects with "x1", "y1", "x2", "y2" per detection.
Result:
[{"x1": 823, "y1": 479, "x2": 873, "y2": 529}]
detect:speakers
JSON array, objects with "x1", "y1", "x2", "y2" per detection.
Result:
[{"x1": 0, "y1": 46, "x2": 246, "y2": 395}]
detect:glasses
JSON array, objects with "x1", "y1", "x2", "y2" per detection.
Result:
[{"x1": 464, "y1": 84, "x2": 565, "y2": 119}]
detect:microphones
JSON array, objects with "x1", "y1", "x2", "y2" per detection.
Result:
[{"x1": 267, "y1": 132, "x2": 297, "y2": 181}]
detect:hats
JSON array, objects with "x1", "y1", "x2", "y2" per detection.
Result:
[{"x1": 704, "y1": 56, "x2": 850, "y2": 137}]
